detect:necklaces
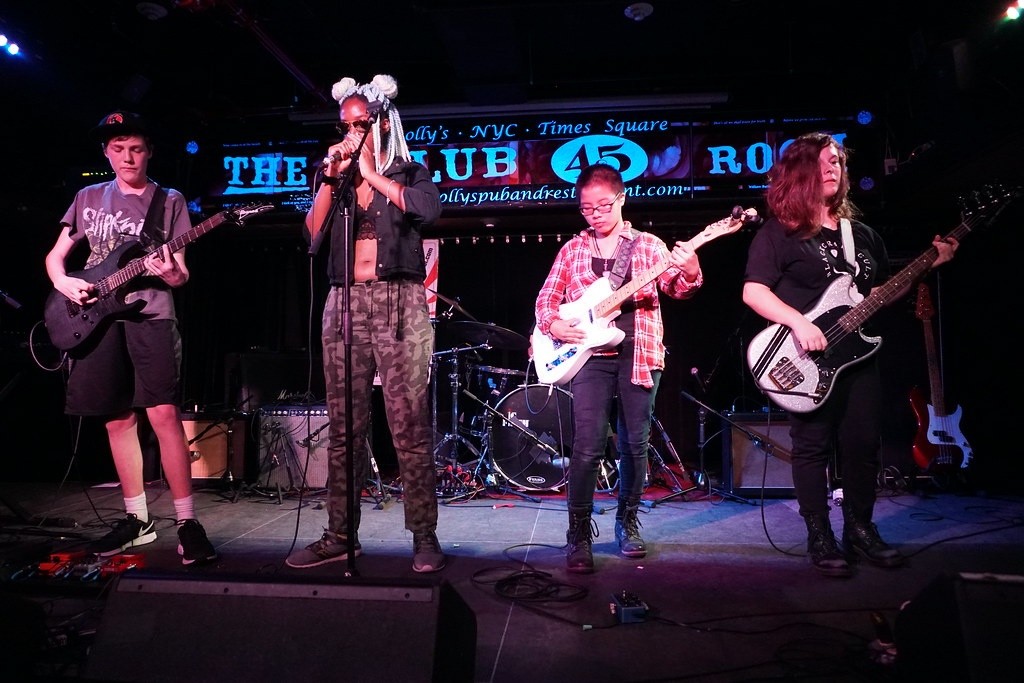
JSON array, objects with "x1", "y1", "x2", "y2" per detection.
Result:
[
  {"x1": 591, "y1": 230, "x2": 625, "y2": 279},
  {"x1": 812, "y1": 216, "x2": 841, "y2": 258}
]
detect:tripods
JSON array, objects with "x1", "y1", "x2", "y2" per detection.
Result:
[
  {"x1": 444, "y1": 389, "x2": 557, "y2": 507},
  {"x1": 655, "y1": 391, "x2": 762, "y2": 505},
  {"x1": 187, "y1": 392, "x2": 254, "y2": 500},
  {"x1": 389, "y1": 332, "x2": 493, "y2": 482}
]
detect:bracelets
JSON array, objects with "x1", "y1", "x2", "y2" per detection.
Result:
[{"x1": 385, "y1": 179, "x2": 396, "y2": 206}]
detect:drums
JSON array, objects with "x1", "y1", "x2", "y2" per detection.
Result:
[
  {"x1": 459, "y1": 363, "x2": 535, "y2": 438},
  {"x1": 488, "y1": 383, "x2": 576, "y2": 491}
]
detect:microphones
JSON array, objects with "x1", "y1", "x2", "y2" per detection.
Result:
[
  {"x1": 189, "y1": 451, "x2": 201, "y2": 458},
  {"x1": 296, "y1": 439, "x2": 315, "y2": 448},
  {"x1": 733, "y1": 205, "x2": 749, "y2": 219},
  {"x1": 692, "y1": 367, "x2": 706, "y2": 393},
  {"x1": 319, "y1": 153, "x2": 342, "y2": 171},
  {"x1": 552, "y1": 452, "x2": 571, "y2": 469}
]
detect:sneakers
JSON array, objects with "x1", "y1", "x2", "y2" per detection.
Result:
[
  {"x1": 286, "y1": 526, "x2": 362, "y2": 568},
  {"x1": 412, "y1": 530, "x2": 445, "y2": 572},
  {"x1": 85, "y1": 514, "x2": 158, "y2": 557},
  {"x1": 166, "y1": 518, "x2": 218, "y2": 565}
]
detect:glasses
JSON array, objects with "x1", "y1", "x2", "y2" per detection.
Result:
[
  {"x1": 335, "y1": 121, "x2": 372, "y2": 134},
  {"x1": 578, "y1": 192, "x2": 620, "y2": 216}
]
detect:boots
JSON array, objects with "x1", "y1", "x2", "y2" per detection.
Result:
[
  {"x1": 566, "y1": 503, "x2": 599, "y2": 572},
  {"x1": 804, "y1": 512, "x2": 851, "y2": 575},
  {"x1": 615, "y1": 495, "x2": 650, "y2": 556},
  {"x1": 841, "y1": 499, "x2": 904, "y2": 567}
]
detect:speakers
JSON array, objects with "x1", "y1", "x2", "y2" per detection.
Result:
[
  {"x1": 258, "y1": 404, "x2": 332, "y2": 490},
  {"x1": 177, "y1": 411, "x2": 253, "y2": 482},
  {"x1": 80, "y1": 573, "x2": 478, "y2": 683},
  {"x1": 893, "y1": 568, "x2": 1024, "y2": 683},
  {"x1": 721, "y1": 412, "x2": 832, "y2": 496}
]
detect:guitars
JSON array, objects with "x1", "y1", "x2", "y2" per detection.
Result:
[
  {"x1": 44, "y1": 199, "x2": 278, "y2": 357},
  {"x1": 744, "y1": 177, "x2": 1023, "y2": 415},
  {"x1": 905, "y1": 281, "x2": 977, "y2": 472},
  {"x1": 531, "y1": 206, "x2": 760, "y2": 387}
]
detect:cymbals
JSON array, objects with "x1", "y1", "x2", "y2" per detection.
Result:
[
  {"x1": 426, "y1": 287, "x2": 478, "y2": 322},
  {"x1": 448, "y1": 320, "x2": 532, "y2": 350}
]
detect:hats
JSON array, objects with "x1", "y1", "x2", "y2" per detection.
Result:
[{"x1": 89, "y1": 111, "x2": 152, "y2": 145}]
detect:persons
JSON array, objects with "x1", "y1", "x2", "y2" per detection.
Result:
[
  {"x1": 741, "y1": 132, "x2": 962, "y2": 568},
  {"x1": 535, "y1": 164, "x2": 704, "y2": 575},
  {"x1": 285, "y1": 73, "x2": 449, "y2": 574},
  {"x1": 45, "y1": 110, "x2": 223, "y2": 565}
]
